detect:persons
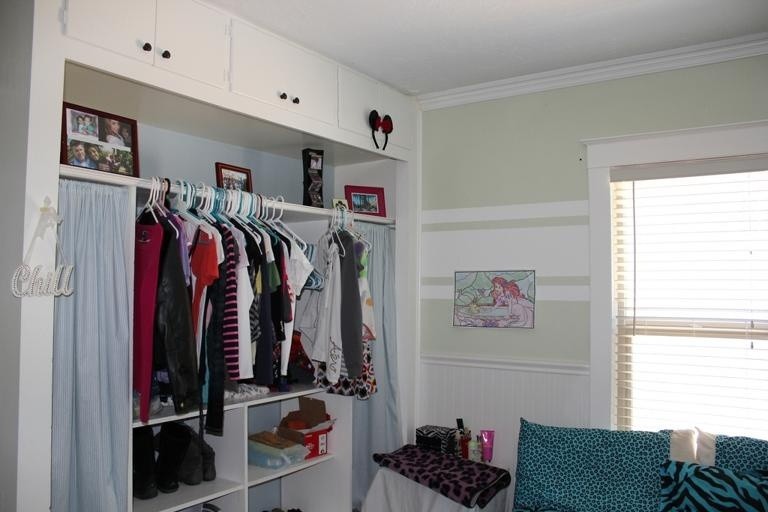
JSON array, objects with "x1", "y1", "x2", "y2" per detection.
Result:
[
  {"x1": 491, "y1": 277, "x2": 534, "y2": 327},
  {"x1": 70, "y1": 114, "x2": 132, "y2": 171}
]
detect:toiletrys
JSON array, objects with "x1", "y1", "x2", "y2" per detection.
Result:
[
  {"x1": 460, "y1": 437, "x2": 472, "y2": 459},
  {"x1": 468, "y1": 440, "x2": 482, "y2": 463}
]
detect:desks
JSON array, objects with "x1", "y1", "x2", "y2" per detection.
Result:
[{"x1": 360, "y1": 462, "x2": 511, "y2": 512}]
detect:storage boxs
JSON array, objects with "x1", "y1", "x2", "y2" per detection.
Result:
[
  {"x1": 281, "y1": 397, "x2": 332, "y2": 457},
  {"x1": 249, "y1": 431, "x2": 311, "y2": 469}
]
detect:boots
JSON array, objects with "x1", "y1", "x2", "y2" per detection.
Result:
[{"x1": 133, "y1": 420, "x2": 220, "y2": 500}]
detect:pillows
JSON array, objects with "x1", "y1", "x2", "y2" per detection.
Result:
[
  {"x1": 514, "y1": 416, "x2": 694, "y2": 512},
  {"x1": 669, "y1": 429, "x2": 697, "y2": 463},
  {"x1": 694, "y1": 427, "x2": 768, "y2": 470},
  {"x1": 659, "y1": 459, "x2": 768, "y2": 512},
  {"x1": 696, "y1": 427, "x2": 715, "y2": 466}
]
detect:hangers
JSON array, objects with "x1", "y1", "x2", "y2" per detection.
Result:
[
  {"x1": 136, "y1": 176, "x2": 307, "y2": 250},
  {"x1": 321, "y1": 205, "x2": 373, "y2": 257},
  {"x1": 300, "y1": 243, "x2": 326, "y2": 290}
]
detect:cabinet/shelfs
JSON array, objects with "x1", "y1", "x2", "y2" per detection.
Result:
[
  {"x1": 54, "y1": 165, "x2": 394, "y2": 512},
  {"x1": 339, "y1": 67, "x2": 418, "y2": 167},
  {"x1": 232, "y1": 17, "x2": 337, "y2": 166},
  {"x1": 66, "y1": 1, "x2": 228, "y2": 143}
]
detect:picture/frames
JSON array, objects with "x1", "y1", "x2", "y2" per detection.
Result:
[
  {"x1": 215, "y1": 162, "x2": 252, "y2": 192},
  {"x1": 62, "y1": 102, "x2": 139, "y2": 178},
  {"x1": 345, "y1": 185, "x2": 386, "y2": 217}
]
